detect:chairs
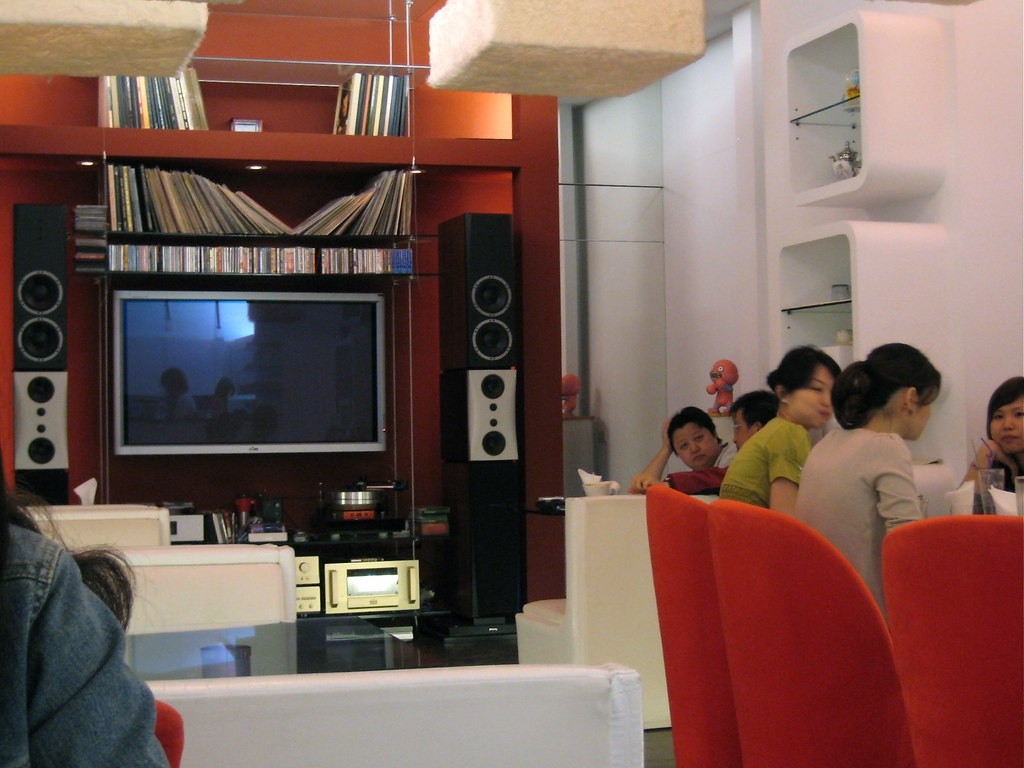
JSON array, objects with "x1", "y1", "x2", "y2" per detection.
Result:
[
  {"x1": 645, "y1": 480, "x2": 741, "y2": 768},
  {"x1": 880, "y1": 514, "x2": 1024, "y2": 768},
  {"x1": 706, "y1": 497, "x2": 921, "y2": 768}
]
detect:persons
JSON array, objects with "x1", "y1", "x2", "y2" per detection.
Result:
[
  {"x1": 719, "y1": 344, "x2": 843, "y2": 517},
  {"x1": 950, "y1": 376, "x2": 1024, "y2": 517},
  {"x1": 211, "y1": 376, "x2": 236, "y2": 415},
  {"x1": 631, "y1": 388, "x2": 780, "y2": 496},
  {"x1": 161, "y1": 368, "x2": 197, "y2": 422},
  {"x1": 0, "y1": 449, "x2": 173, "y2": 768},
  {"x1": 792, "y1": 343, "x2": 942, "y2": 618}
]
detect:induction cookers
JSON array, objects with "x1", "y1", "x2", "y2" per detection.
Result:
[{"x1": 322, "y1": 509, "x2": 394, "y2": 540}]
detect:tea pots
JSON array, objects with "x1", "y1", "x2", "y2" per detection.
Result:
[{"x1": 828, "y1": 141, "x2": 861, "y2": 179}]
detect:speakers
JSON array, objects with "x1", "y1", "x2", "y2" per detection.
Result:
[{"x1": 438, "y1": 213, "x2": 527, "y2": 627}]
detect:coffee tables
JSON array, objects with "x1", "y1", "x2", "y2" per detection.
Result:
[{"x1": 122, "y1": 608, "x2": 520, "y2": 681}]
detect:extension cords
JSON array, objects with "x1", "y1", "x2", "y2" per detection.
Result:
[{"x1": 9, "y1": 202, "x2": 69, "y2": 508}]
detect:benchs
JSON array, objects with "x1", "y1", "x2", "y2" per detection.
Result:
[
  {"x1": 514, "y1": 492, "x2": 723, "y2": 732},
  {"x1": 18, "y1": 505, "x2": 173, "y2": 558},
  {"x1": 141, "y1": 663, "x2": 645, "y2": 768},
  {"x1": 114, "y1": 543, "x2": 296, "y2": 636}
]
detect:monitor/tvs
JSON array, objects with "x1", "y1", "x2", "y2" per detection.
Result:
[{"x1": 113, "y1": 290, "x2": 388, "y2": 453}]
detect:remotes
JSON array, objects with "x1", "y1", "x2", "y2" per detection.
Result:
[{"x1": 536, "y1": 495, "x2": 564, "y2": 509}]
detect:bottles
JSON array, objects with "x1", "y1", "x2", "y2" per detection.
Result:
[{"x1": 841, "y1": 70, "x2": 861, "y2": 113}]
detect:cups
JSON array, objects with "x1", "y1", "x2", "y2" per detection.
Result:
[
  {"x1": 582, "y1": 483, "x2": 610, "y2": 496},
  {"x1": 978, "y1": 469, "x2": 1004, "y2": 515},
  {"x1": 1015, "y1": 476, "x2": 1024, "y2": 516}
]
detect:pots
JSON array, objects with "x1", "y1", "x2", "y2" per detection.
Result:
[{"x1": 329, "y1": 489, "x2": 380, "y2": 511}]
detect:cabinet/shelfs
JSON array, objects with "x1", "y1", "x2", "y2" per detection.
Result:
[{"x1": 173, "y1": 527, "x2": 452, "y2": 620}]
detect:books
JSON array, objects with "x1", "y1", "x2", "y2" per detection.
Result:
[
  {"x1": 199, "y1": 508, "x2": 235, "y2": 544},
  {"x1": 107, "y1": 164, "x2": 412, "y2": 235},
  {"x1": 332, "y1": 72, "x2": 409, "y2": 137},
  {"x1": 98, "y1": 67, "x2": 209, "y2": 132}
]
